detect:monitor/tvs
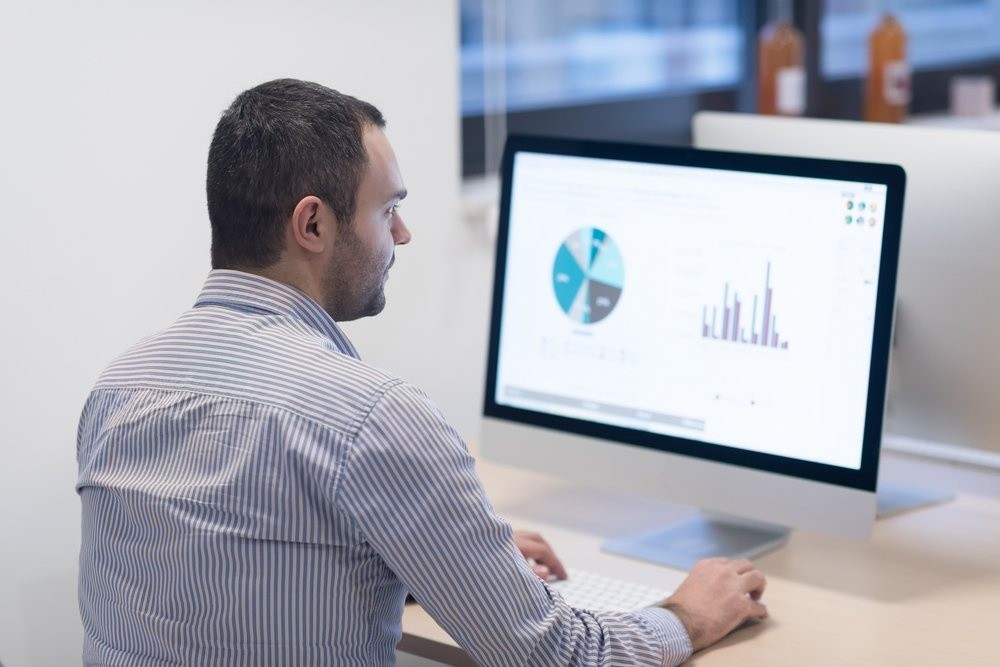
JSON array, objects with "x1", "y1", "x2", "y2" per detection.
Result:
[
  {"x1": 690, "y1": 108, "x2": 1000, "y2": 498},
  {"x1": 479, "y1": 131, "x2": 907, "y2": 542}
]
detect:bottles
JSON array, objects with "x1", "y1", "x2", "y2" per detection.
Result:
[
  {"x1": 871, "y1": 11, "x2": 911, "y2": 123},
  {"x1": 757, "y1": 15, "x2": 807, "y2": 117}
]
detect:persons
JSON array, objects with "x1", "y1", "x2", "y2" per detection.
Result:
[{"x1": 74, "y1": 76, "x2": 767, "y2": 665}]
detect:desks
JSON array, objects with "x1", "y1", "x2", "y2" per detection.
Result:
[{"x1": 399, "y1": 396, "x2": 1000, "y2": 666}]
